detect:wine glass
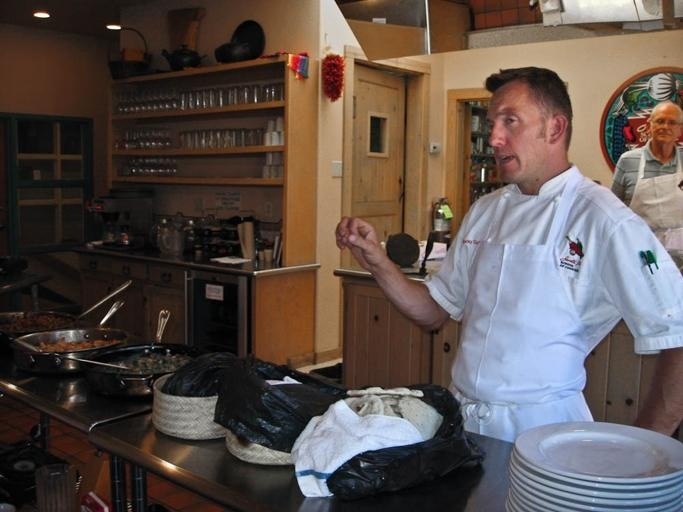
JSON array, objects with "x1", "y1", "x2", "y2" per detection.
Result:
[{"x1": 110, "y1": 85, "x2": 187, "y2": 152}]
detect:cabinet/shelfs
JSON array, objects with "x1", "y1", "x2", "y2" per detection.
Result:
[
  {"x1": 433, "y1": 315, "x2": 460, "y2": 389},
  {"x1": 583, "y1": 317, "x2": 641, "y2": 426},
  {"x1": 339, "y1": 276, "x2": 432, "y2": 389},
  {"x1": 106, "y1": 53, "x2": 318, "y2": 268},
  {"x1": 78, "y1": 253, "x2": 185, "y2": 344},
  {"x1": 462, "y1": 101, "x2": 501, "y2": 217}
]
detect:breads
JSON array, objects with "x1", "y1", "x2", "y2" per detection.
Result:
[{"x1": 344, "y1": 386, "x2": 445, "y2": 442}]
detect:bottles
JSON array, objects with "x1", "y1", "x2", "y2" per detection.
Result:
[
  {"x1": 260, "y1": 151, "x2": 284, "y2": 182},
  {"x1": 155, "y1": 216, "x2": 182, "y2": 256},
  {"x1": 469, "y1": 103, "x2": 502, "y2": 201},
  {"x1": 194, "y1": 244, "x2": 204, "y2": 256},
  {"x1": 119, "y1": 224, "x2": 130, "y2": 245},
  {"x1": 97, "y1": 210, "x2": 118, "y2": 244},
  {"x1": 181, "y1": 220, "x2": 199, "y2": 255}
]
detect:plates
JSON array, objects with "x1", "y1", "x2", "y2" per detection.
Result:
[{"x1": 501, "y1": 419, "x2": 683, "y2": 512}]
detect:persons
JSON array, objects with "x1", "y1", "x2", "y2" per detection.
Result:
[
  {"x1": 335, "y1": 67, "x2": 682, "y2": 444},
  {"x1": 612, "y1": 100, "x2": 683, "y2": 258}
]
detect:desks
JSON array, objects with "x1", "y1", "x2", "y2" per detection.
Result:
[
  {"x1": 87, "y1": 413, "x2": 514, "y2": 512},
  {"x1": 0, "y1": 355, "x2": 152, "y2": 452}
]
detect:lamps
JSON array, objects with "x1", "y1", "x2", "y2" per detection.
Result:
[{"x1": 105, "y1": 21, "x2": 149, "y2": 58}]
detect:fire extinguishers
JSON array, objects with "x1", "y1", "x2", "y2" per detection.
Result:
[{"x1": 432, "y1": 198, "x2": 451, "y2": 248}]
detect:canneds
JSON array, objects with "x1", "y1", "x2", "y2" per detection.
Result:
[{"x1": 201, "y1": 222, "x2": 240, "y2": 258}]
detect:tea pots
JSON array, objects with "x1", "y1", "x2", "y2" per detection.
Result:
[{"x1": 162, "y1": 44, "x2": 201, "y2": 69}]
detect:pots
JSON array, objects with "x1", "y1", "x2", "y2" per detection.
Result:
[
  {"x1": 75, "y1": 307, "x2": 206, "y2": 403},
  {"x1": 0, "y1": 279, "x2": 133, "y2": 341},
  {"x1": 7, "y1": 301, "x2": 131, "y2": 373}
]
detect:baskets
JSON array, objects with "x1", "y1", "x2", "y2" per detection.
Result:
[
  {"x1": 151, "y1": 373, "x2": 227, "y2": 440},
  {"x1": 225, "y1": 427, "x2": 293, "y2": 466}
]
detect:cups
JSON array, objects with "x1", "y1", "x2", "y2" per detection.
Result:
[
  {"x1": 178, "y1": 114, "x2": 284, "y2": 149},
  {"x1": 180, "y1": 82, "x2": 285, "y2": 111},
  {"x1": 126, "y1": 157, "x2": 178, "y2": 175}
]
